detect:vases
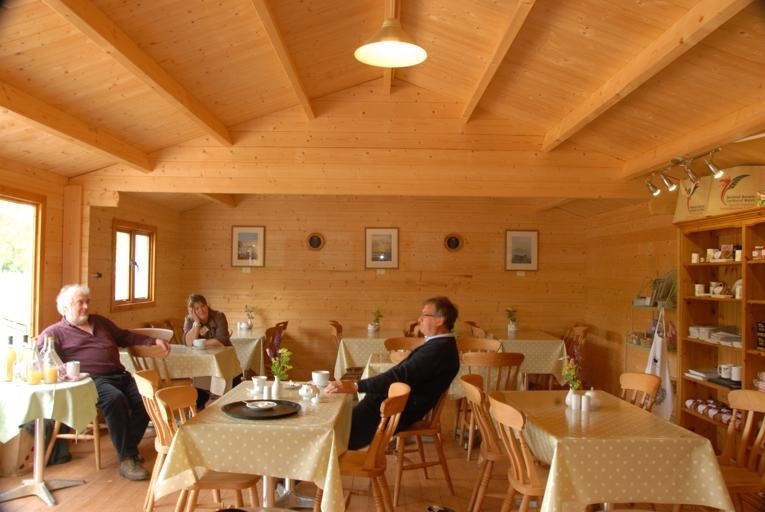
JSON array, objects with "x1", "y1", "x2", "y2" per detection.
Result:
[
  {"x1": 271, "y1": 376, "x2": 279, "y2": 400},
  {"x1": 238, "y1": 319, "x2": 252, "y2": 330},
  {"x1": 565, "y1": 383, "x2": 578, "y2": 407}
]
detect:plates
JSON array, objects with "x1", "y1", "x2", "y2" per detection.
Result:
[
  {"x1": 283, "y1": 381, "x2": 303, "y2": 388},
  {"x1": 220, "y1": 399, "x2": 301, "y2": 420},
  {"x1": 247, "y1": 401, "x2": 277, "y2": 410}
]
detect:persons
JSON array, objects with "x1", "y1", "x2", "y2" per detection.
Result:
[
  {"x1": 276, "y1": 297, "x2": 459, "y2": 487},
  {"x1": 183, "y1": 294, "x2": 242, "y2": 414},
  {"x1": 37, "y1": 284, "x2": 171, "y2": 481}
]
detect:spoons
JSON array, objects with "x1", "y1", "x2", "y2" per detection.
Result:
[{"x1": 240, "y1": 399, "x2": 259, "y2": 408}]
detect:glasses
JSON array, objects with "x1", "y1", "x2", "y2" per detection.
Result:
[{"x1": 420, "y1": 312, "x2": 437, "y2": 319}]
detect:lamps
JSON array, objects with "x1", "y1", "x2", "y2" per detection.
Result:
[
  {"x1": 680, "y1": 156, "x2": 700, "y2": 184},
  {"x1": 352, "y1": 16, "x2": 428, "y2": 68},
  {"x1": 704, "y1": 151, "x2": 724, "y2": 179},
  {"x1": 659, "y1": 169, "x2": 676, "y2": 192},
  {"x1": 645, "y1": 174, "x2": 661, "y2": 197}
]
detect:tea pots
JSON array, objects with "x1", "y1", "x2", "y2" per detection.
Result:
[
  {"x1": 584, "y1": 387, "x2": 601, "y2": 412},
  {"x1": 240, "y1": 322, "x2": 249, "y2": 331},
  {"x1": 298, "y1": 384, "x2": 320, "y2": 404}
]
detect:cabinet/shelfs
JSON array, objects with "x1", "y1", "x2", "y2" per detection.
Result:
[
  {"x1": 624, "y1": 301, "x2": 678, "y2": 421},
  {"x1": 677, "y1": 205, "x2": 765, "y2": 512}
]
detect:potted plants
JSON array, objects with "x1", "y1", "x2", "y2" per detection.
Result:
[
  {"x1": 504, "y1": 308, "x2": 518, "y2": 337},
  {"x1": 367, "y1": 309, "x2": 383, "y2": 339}
]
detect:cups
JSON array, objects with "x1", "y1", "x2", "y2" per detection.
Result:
[
  {"x1": 237, "y1": 322, "x2": 240, "y2": 330},
  {"x1": 694, "y1": 284, "x2": 706, "y2": 297},
  {"x1": 312, "y1": 370, "x2": 330, "y2": 388},
  {"x1": 735, "y1": 286, "x2": 744, "y2": 299},
  {"x1": 581, "y1": 395, "x2": 591, "y2": 412},
  {"x1": 66, "y1": 361, "x2": 81, "y2": 380},
  {"x1": 731, "y1": 366, "x2": 743, "y2": 382},
  {"x1": 252, "y1": 376, "x2": 267, "y2": 393},
  {"x1": 572, "y1": 394, "x2": 581, "y2": 411},
  {"x1": 691, "y1": 252, "x2": 699, "y2": 264},
  {"x1": 193, "y1": 339, "x2": 208, "y2": 348},
  {"x1": 718, "y1": 362, "x2": 733, "y2": 378},
  {"x1": 263, "y1": 385, "x2": 269, "y2": 400}
]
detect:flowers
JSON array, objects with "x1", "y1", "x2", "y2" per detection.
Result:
[
  {"x1": 266, "y1": 336, "x2": 292, "y2": 380},
  {"x1": 241, "y1": 304, "x2": 266, "y2": 321},
  {"x1": 559, "y1": 355, "x2": 582, "y2": 389}
]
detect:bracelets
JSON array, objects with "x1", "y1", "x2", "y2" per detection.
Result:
[{"x1": 192, "y1": 322, "x2": 202, "y2": 328}]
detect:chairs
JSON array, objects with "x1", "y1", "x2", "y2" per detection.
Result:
[
  {"x1": 619, "y1": 373, "x2": 662, "y2": 413},
  {"x1": 329, "y1": 319, "x2": 362, "y2": 380},
  {"x1": 384, "y1": 336, "x2": 423, "y2": 353},
  {"x1": 458, "y1": 352, "x2": 525, "y2": 462},
  {"x1": 404, "y1": 321, "x2": 421, "y2": 336},
  {"x1": 487, "y1": 393, "x2": 552, "y2": 512},
  {"x1": 245, "y1": 325, "x2": 283, "y2": 379},
  {"x1": 684, "y1": 390, "x2": 765, "y2": 512},
  {"x1": 535, "y1": 326, "x2": 591, "y2": 390},
  {"x1": 312, "y1": 382, "x2": 411, "y2": 512},
  {"x1": 167, "y1": 317, "x2": 185, "y2": 344},
  {"x1": 32, "y1": 336, "x2": 108, "y2": 470},
  {"x1": 393, "y1": 386, "x2": 454, "y2": 506},
  {"x1": 127, "y1": 328, "x2": 174, "y2": 343},
  {"x1": 464, "y1": 321, "x2": 485, "y2": 338},
  {"x1": 149, "y1": 319, "x2": 170, "y2": 329},
  {"x1": 127, "y1": 345, "x2": 192, "y2": 386},
  {"x1": 133, "y1": 370, "x2": 171, "y2": 512},
  {"x1": 154, "y1": 384, "x2": 261, "y2": 512},
  {"x1": 452, "y1": 337, "x2": 504, "y2": 436},
  {"x1": 459, "y1": 374, "x2": 541, "y2": 512},
  {"x1": 275, "y1": 320, "x2": 288, "y2": 329}
]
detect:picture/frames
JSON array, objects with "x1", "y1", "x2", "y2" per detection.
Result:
[
  {"x1": 650, "y1": 278, "x2": 671, "y2": 307},
  {"x1": 364, "y1": 227, "x2": 399, "y2": 269},
  {"x1": 231, "y1": 226, "x2": 265, "y2": 267},
  {"x1": 504, "y1": 230, "x2": 539, "y2": 271}
]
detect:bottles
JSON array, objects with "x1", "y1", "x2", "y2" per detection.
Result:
[
  {"x1": 18, "y1": 335, "x2": 28, "y2": 377},
  {"x1": 43, "y1": 337, "x2": 57, "y2": 384},
  {"x1": 40, "y1": 333, "x2": 49, "y2": 358},
  {"x1": 4, "y1": 336, "x2": 17, "y2": 382},
  {"x1": 26, "y1": 338, "x2": 42, "y2": 384}
]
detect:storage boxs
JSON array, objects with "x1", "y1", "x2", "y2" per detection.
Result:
[
  {"x1": 673, "y1": 176, "x2": 712, "y2": 224},
  {"x1": 705, "y1": 166, "x2": 765, "y2": 216}
]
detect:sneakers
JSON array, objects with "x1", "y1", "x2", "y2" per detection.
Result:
[
  {"x1": 132, "y1": 454, "x2": 145, "y2": 464},
  {"x1": 119, "y1": 459, "x2": 148, "y2": 480}
]
